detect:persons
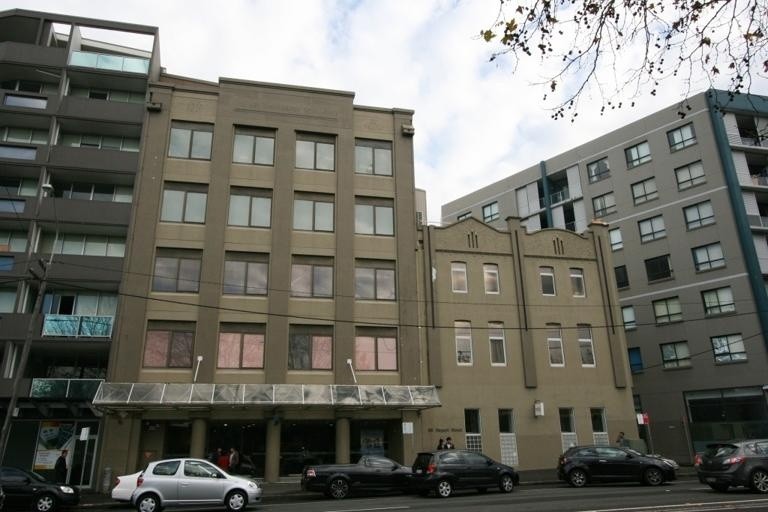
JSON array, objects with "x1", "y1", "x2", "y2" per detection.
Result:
[
  {"x1": 443, "y1": 436, "x2": 455, "y2": 449},
  {"x1": 217, "y1": 450, "x2": 231, "y2": 472},
  {"x1": 436, "y1": 439, "x2": 445, "y2": 450},
  {"x1": 615, "y1": 431, "x2": 629, "y2": 446},
  {"x1": 228, "y1": 446, "x2": 241, "y2": 475},
  {"x1": 53, "y1": 449, "x2": 69, "y2": 485}
]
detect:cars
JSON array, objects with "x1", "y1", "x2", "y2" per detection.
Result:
[
  {"x1": 110, "y1": 469, "x2": 145, "y2": 503},
  {"x1": 210, "y1": 450, "x2": 261, "y2": 475},
  {"x1": 130, "y1": 457, "x2": 263, "y2": 512},
  {"x1": 0, "y1": 464, "x2": 81, "y2": 512}
]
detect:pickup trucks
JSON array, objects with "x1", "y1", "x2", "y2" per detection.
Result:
[{"x1": 299, "y1": 454, "x2": 413, "y2": 501}]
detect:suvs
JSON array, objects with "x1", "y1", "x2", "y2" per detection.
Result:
[
  {"x1": 693, "y1": 438, "x2": 767, "y2": 495},
  {"x1": 410, "y1": 447, "x2": 519, "y2": 500},
  {"x1": 553, "y1": 443, "x2": 679, "y2": 492}
]
